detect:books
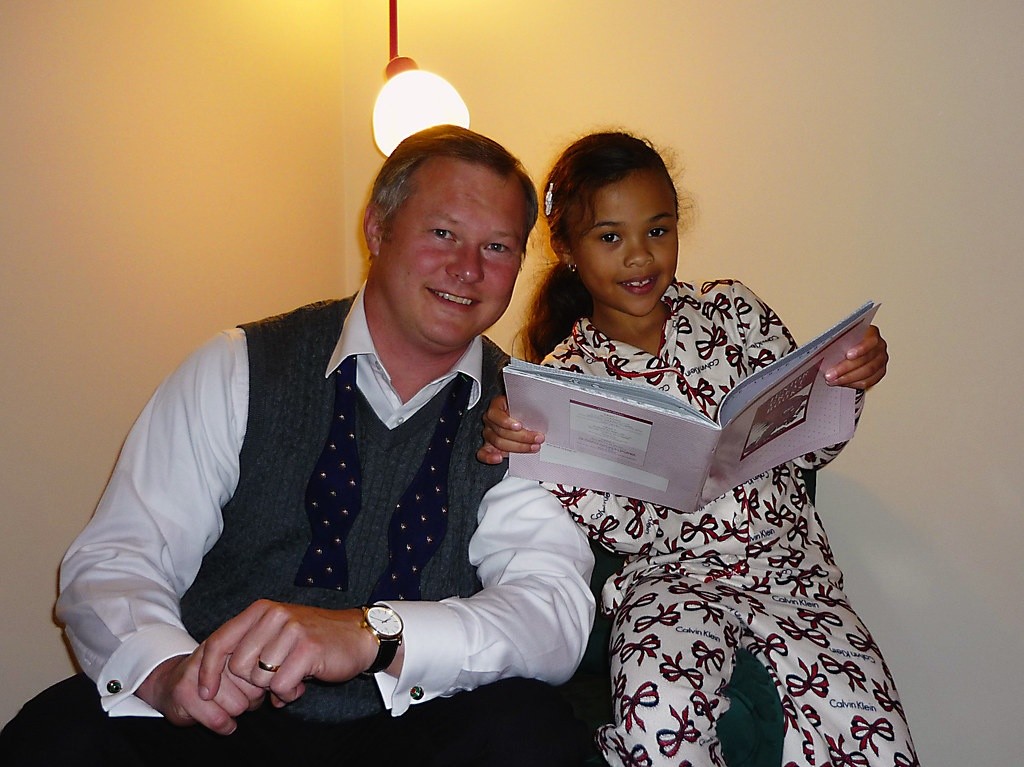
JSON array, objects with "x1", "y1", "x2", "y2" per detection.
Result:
[{"x1": 502, "y1": 300, "x2": 882, "y2": 513}]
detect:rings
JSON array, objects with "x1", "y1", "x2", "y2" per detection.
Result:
[{"x1": 257, "y1": 655, "x2": 282, "y2": 672}]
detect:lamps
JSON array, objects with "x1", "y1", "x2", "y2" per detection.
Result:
[{"x1": 372, "y1": 0, "x2": 469, "y2": 157}]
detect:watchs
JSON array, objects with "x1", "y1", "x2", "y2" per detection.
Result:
[{"x1": 360, "y1": 605, "x2": 404, "y2": 674}]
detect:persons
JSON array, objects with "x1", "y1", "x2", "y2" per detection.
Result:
[
  {"x1": 476, "y1": 132, "x2": 922, "y2": 767},
  {"x1": 0, "y1": 124, "x2": 589, "y2": 767}
]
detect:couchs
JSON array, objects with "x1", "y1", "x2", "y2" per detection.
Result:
[{"x1": 560, "y1": 471, "x2": 817, "y2": 767}]
